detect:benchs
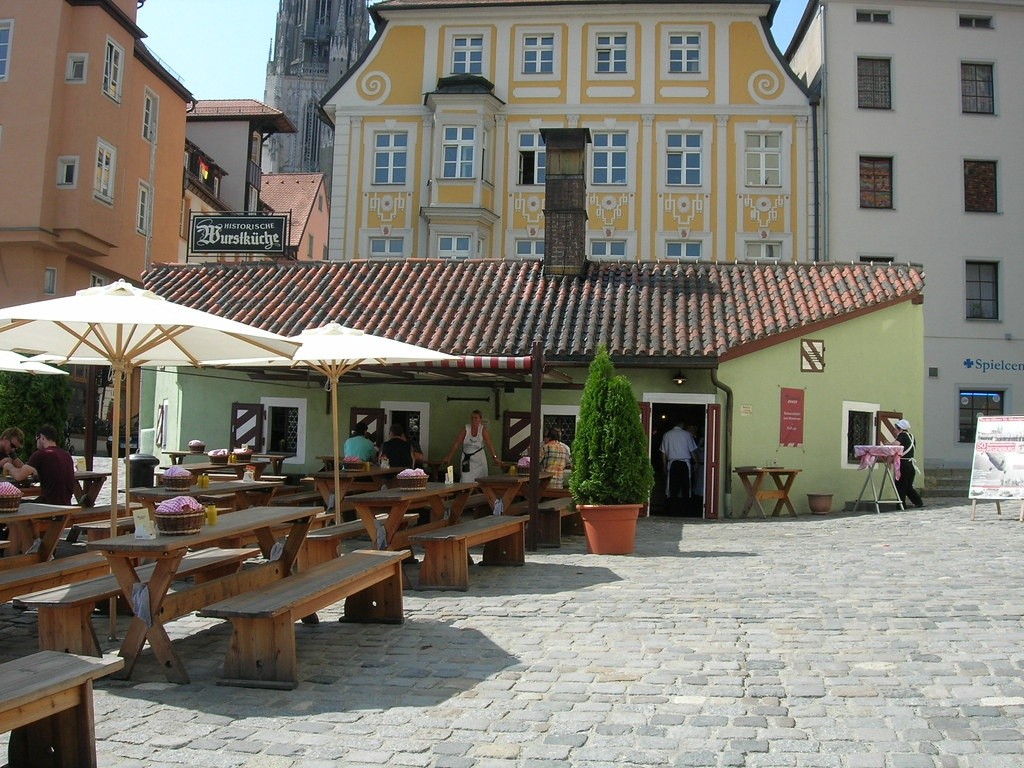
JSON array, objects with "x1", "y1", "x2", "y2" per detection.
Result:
[{"x1": 0, "y1": 473, "x2": 581, "y2": 768}]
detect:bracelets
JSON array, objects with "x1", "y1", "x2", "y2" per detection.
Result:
[{"x1": 493, "y1": 455, "x2": 497, "y2": 458}]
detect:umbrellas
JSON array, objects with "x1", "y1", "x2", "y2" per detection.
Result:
[
  {"x1": 196, "y1": 319, "x2": 462, "y2": 556},
  {"x1": 0, "y1": 279, "x2": 302, "y2": 636}
]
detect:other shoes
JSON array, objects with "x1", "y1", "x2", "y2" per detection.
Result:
[
  {"x1": 681, "y1": 511, "x2": 689, "y2": 515},
  {"x1": 898, "y1": 503, "x2": 906, "y2": 509},
  {"x1": 913, "y1": 502, "x2": 923, "y2": 507},
  {"x1": 672, "y1": 512, "x2": 680, "y2": 517}
]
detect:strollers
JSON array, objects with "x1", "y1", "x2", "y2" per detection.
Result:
[{"x1": 63, "y1": 420, "x2": 75, "y2": 455}]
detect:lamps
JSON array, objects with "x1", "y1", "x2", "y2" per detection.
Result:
[{"x1": 673, "y1": 368, "x2": 688, "y2": 384}]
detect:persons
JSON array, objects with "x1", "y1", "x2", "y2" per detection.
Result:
[
  {"x1": 660, "y1": 415, "x2": 698, "y2": 516},
  {"x1": 539, "y1": 426, "x2": 572, "y2": 488},
  {"x1": 4, "y1": 426, "x2": 74, "y2": 560},
  {"x1": 443, "y1": 410, "x2": 500, "y2": 483},
  {"x1": 0, "y1": 428, "x2": 24, "y2": 474},
  {"x1": 344, "y1": 422, "x2": 424, "y2": 476},
  {"x1": 883, "y1": 420, "x2": 922, "y2": 508}
]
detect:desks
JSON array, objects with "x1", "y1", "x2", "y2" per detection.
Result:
[
  {"x1": 851, "y1": 444, "x2": 904, "y2": 516},
  {"x1": 86, "y1": 506, "x2": 325, "y2": 688},
  {"x1": 474, "y1": 470, "x2": 556, "y2": 516},
  {"x1": 161, "y1": 451, "x2": 293, "y2": 476},
  {"x1": 0, "y1": 470, "x2": 112, "y2": 543},
  {"x1": 0, "y1": 502, "x2": 83, "y2": 609},
  {"x1": 733, "y1": 468, "x2": 803, "y2": 520},
  {"x1": 344, "y1": 482, "x2": 480, "y2": 590},
  {"x1": 158, "y1": 461, "x2": 271, "y2": 477},
  {"x1": 304, "y1": 466, "x2": 410, "y2": 527},
  {"x1": 116, "y1": 480, "x2": 285, "y2": 526}
]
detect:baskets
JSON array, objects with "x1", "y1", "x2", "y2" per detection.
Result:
[
  {"x1": 189, "y1": 445, "x2": 205, "y2": 453},
  {"x1": 397, "y1": 475, "x2": 428, "y2": 491},
  {"x1": 517, "y1": 465, "x2": 529, "y2": 477},
  {"x1": 208, "y1": 454, "x2": 230, "y2": 465},
  {"x1": 234, "y1": 453, "x2": 252, "y2": 463},
  {"x1": 0, "y1": 493, "x2": 23, "y2": 512},
  {"x1": 160, "y1": 475, "x2": 191, "y2": 491},
  {"x1": 343, "y1": 462, "x2": 363, "y2": 472},
  {"x1": 154, "y1": 509, "x2": 206, "y2": 536}
]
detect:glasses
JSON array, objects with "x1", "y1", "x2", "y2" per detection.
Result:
[{"x1": 9, "y1": 440, "x2": 19, "y2": 452}]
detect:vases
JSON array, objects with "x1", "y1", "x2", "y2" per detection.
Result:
[{"x1": 806, "y1": 492, "x2": 834, "y2": 515}]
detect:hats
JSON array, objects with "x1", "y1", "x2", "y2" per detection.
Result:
[{"x1": 896, "y1": 420, "x2": 910, "y2": 429}]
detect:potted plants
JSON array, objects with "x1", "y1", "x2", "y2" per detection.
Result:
[{"x1": 566, "y1": 341, "x2": 655, "y2": 555}]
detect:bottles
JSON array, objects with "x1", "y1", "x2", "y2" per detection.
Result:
[
  {"x1": 361, "y1": 458, "x2": 369, "y2": 471},
  {"x1": 229, "y1": 453, "x2": 236, "y2": 463},
  {"x1": 201, "y1": 504, "x2": 206, "y2": 525},
  {"x1": 197, "y1": 473, "x2": 209, "y2": 488},
  {"x1": 510, "y1": 463, "x2": 516, "y2": 474},
  {"x1": 208, "y1": 501, "x2": 217, "y2": 525}
]
